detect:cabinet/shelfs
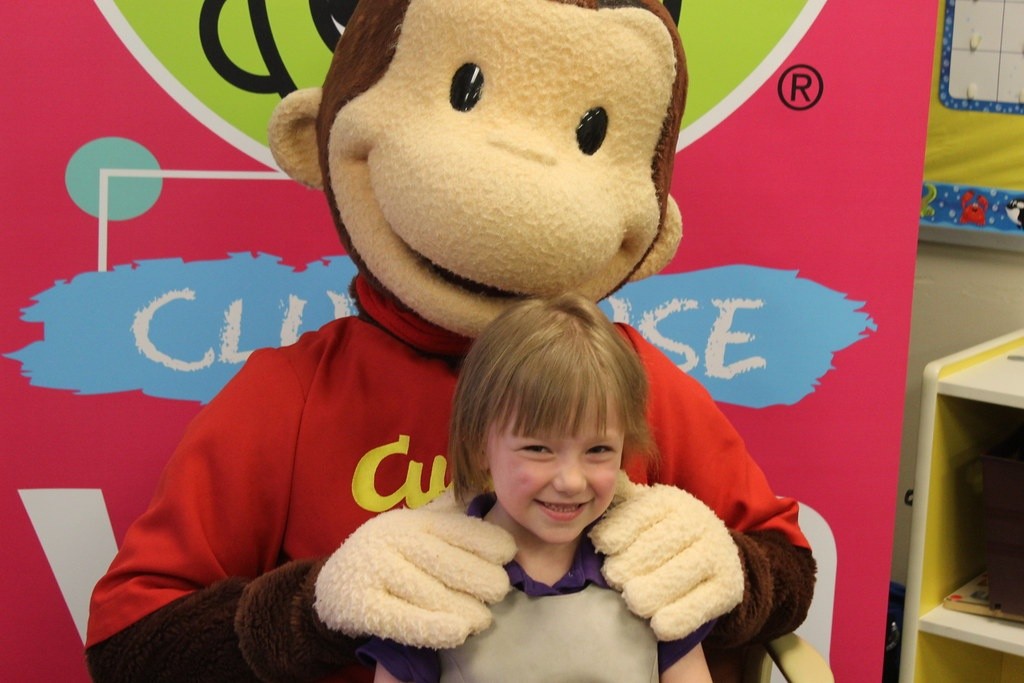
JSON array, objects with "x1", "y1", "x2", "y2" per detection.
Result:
[{"x1": 901, "y1": 325, "x2": 1024, "y2": 683}]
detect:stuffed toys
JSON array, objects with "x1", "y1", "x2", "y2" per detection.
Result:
[{"x1": 84, "y1": 0, "x2": 817, "y2": 682}]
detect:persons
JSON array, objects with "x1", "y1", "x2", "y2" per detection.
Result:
[{"x1": 375, "y1": 292, "x2": 716, "y2": 682}]
